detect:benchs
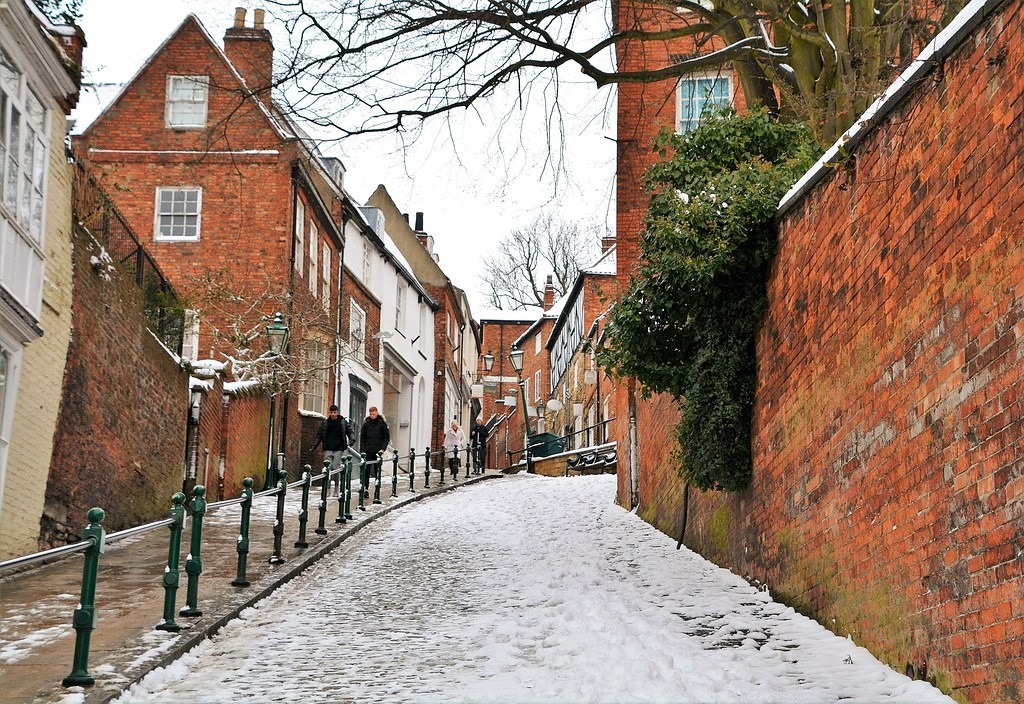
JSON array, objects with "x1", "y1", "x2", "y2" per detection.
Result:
[{"x1": 566, "y1": 445, "x2": 618, "y2": 477}]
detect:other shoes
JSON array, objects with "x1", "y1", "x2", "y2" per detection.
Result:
[
  {"x1": 450, "y1": 473, "x2": 454, "y2": 475},
  {"x1": 333, "y1": 487, "x2": 338, "y2": 497},
  {"x1": 326, "y1": 489, "x2": 331, "y2": 497},
  {"x1": 364, "y1": 491, "x2": 369, "y2": 498},
  {"x1": 471, "y1": 471, "x2": 475, "y2": 474}
]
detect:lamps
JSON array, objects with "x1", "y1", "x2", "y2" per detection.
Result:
[
  {"x1": 570, "y1": 398, "x2": 586, "y2": 416},
  {"x1": 472, "y1": 349, "x2": 496, "y2": 376},
  {"x1": 583, "y1": 368, "x2": 602, "y2": 384}
]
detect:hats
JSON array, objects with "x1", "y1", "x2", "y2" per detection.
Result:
[{"x1": 476, "y1": 417, "x2": 483, "y2": 422}]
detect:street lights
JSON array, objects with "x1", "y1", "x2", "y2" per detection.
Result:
[
  {"x1": 258, "y1": 311, "x2": 290, "y2": 497},
  {"x1": 507, "y1": 342, "x2": 532, "y2": 437}
]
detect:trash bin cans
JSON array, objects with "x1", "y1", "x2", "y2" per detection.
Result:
[{"x1": 528, "y1": 432, "x2": 566, "y2": 457}]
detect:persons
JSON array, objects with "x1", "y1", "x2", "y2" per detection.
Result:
[
  {"x1": 444, "y1": 421, "x2": 466, "y2": 475},
  {"x1": 469, "y1": 416, "x2": 489, "y2": 474},
  {"x1": 309, "y1": 405, "x2": 355, "y2": 497},
  {"x1": 360, "y1": 407, "x2": 390, "y2": 498}
]
